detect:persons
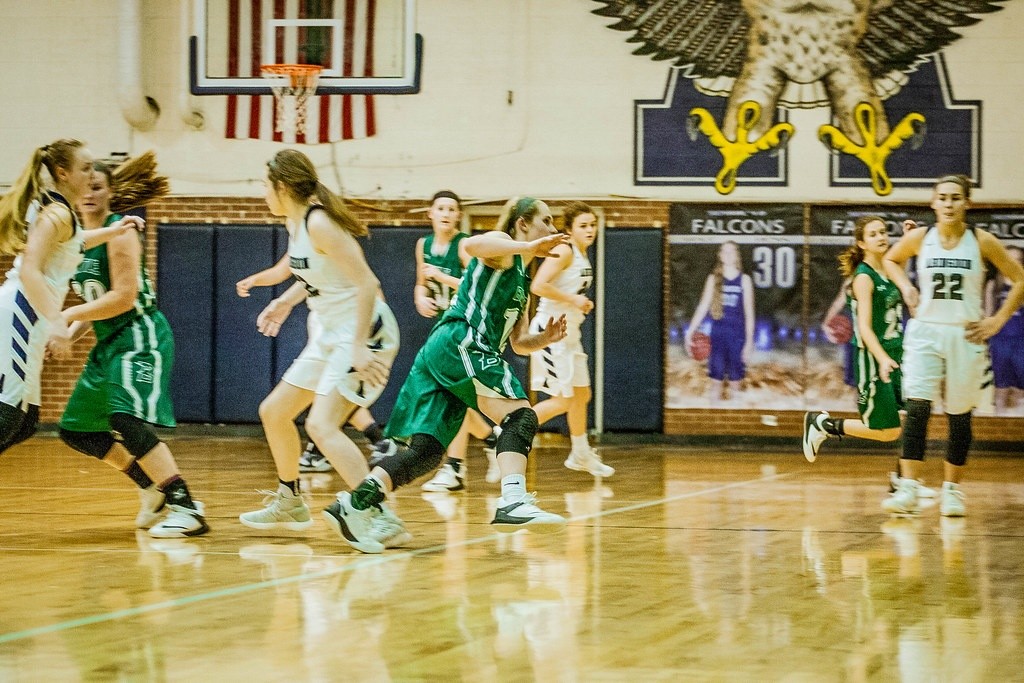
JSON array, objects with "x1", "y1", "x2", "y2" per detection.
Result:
[
  {"x1": 803, "y1": 215, "x2": 937, "y2": 498},
  {"x1": 414, "y1": 190, "x2": 502, "y2": 491},
  {"x1": 235, "y1": 148, "x2": 412, "y2": 551},
  {"x1": 986, "y1": 245, "x2": 1023, "y2": 417},
  {"x1": 494, "y1": 200, "x2": 615, "y2": 478},
  {"x1": 58, "y1": 148, "x2": 211, "y2": 539},
  {"x1": 320, "y1": 196, "x2": 567, "y2": 553},
  {"x1": 882, "y1": 176, "x2": 1024, "y2": 517},
  {"x1": 684, "y1": 240, "x2": 754, "y2": 407},
  {"x1": 821, "y1": 272, "x2": 907, "y2": 400},
  {"x1": 257, "y1": 282, "x2": 400, "y2": 473},
  {"x1": 0, "y1": 138, "x2": 146, "y2": 458}
]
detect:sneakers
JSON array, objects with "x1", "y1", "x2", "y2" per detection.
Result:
[
  {"x1": 940, "y1": 488, "x2": 969, "y2": 516},
  {"x1": 151, "y1": 498, "x2": 212, "y2": 539},
  {"x1": 368, "y1": 440, "x2": 399, "y2": 469},
  {"x1": 483, "y1": 424, "x2": 503, "y2": 484},
  {"x1": 493, "y1": 489, "x2": 567, "y2": 525},
  {"x1": 563, "y1": 446, "x2": 616, "y2": 476},
  {"x1": 134, "y1": 481, "x2": 164, "y2": 527},
  {"x1": 240, "y1": 485, "x2": 314, "y2": 530},
  {"x1": 323, "y1": 490, "x2": 385, "y2": 554},
  {"x1": 802, "y1": 409, "x2": 833, "y2": 462},
  {"x1": 880, "y1": 476, "x2": 923, "y2": 515},
  {"x1": 297, "y1": 440, "x2": 334, "y2": 473},
  {"x1": 887, "y1": 473, "x2": 936, "y2": 498},
  {"x1": 421, "y1": 461, "x2": 468, "y2": 493},
  {"x1": 362, "y1": 505, "x2": 413, "y2": 548}
]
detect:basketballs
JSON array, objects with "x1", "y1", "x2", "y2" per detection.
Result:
[
  {"x1": 692, "y1": 331, "x2": 712, "y2": 361},
  {"x1": 829, "y1": 314, "x2": 853, "y2": 345}
]
